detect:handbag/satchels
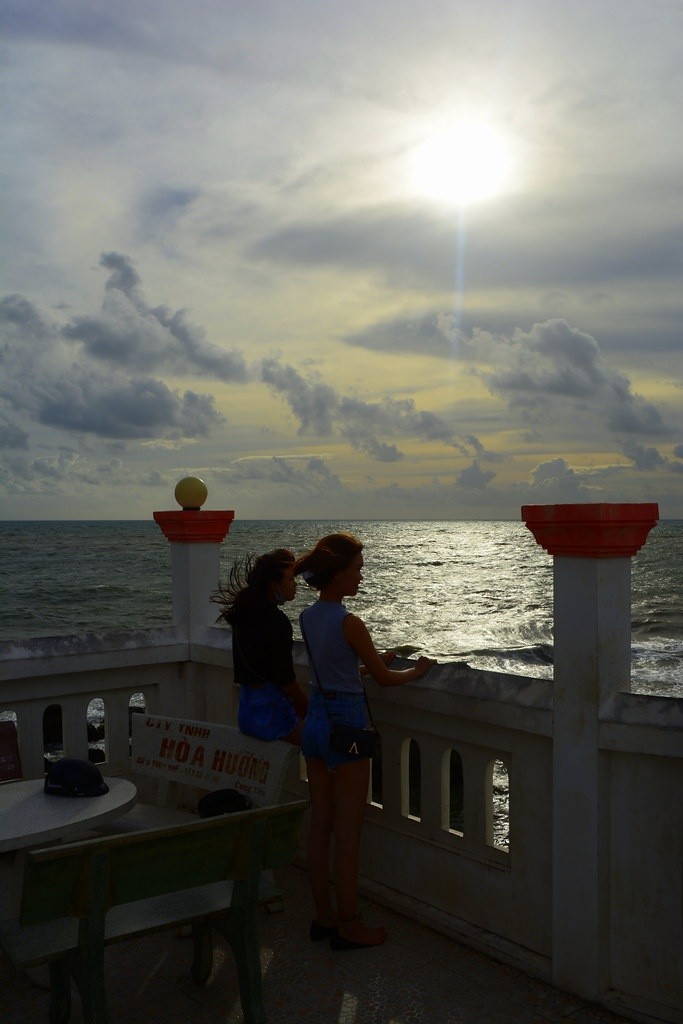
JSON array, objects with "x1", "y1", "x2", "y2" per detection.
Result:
[{"x1": 330, "y1": 723, "x2": 379, "y2": 759}]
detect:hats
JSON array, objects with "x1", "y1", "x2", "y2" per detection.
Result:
[
  {"x1": 44, "y1": 756, "x2": 110, "y2": 797},
  {"x1": 197, "y1": 788, "x2": 252, "y2": 820}
]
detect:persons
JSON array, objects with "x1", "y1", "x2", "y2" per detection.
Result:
[
  {"x1": 209, "y1": 547, "x2": 310, "y2": 911},
  {"x1": 290, "y1": 532, "x2": 437, "y2": 950}
]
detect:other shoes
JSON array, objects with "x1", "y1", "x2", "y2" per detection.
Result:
[
  {"x1": 330, "y1": 934, "x2": 382, "y2": 951},
  {"x1": 309, "y1": 919, "x2": 337, "y2": 942}
]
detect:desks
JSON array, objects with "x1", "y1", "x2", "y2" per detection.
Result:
[{"x1": 0, "y1": 777, "x2": 138, "y2": 852}]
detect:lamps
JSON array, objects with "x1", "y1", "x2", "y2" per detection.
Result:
[{"x1": 175, "y1": 477, "x2": 208, "y2": 510}]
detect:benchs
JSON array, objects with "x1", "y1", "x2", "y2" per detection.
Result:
[{"x1": 0, "y1": 712, "x2": 307, "y2": 1024}]
